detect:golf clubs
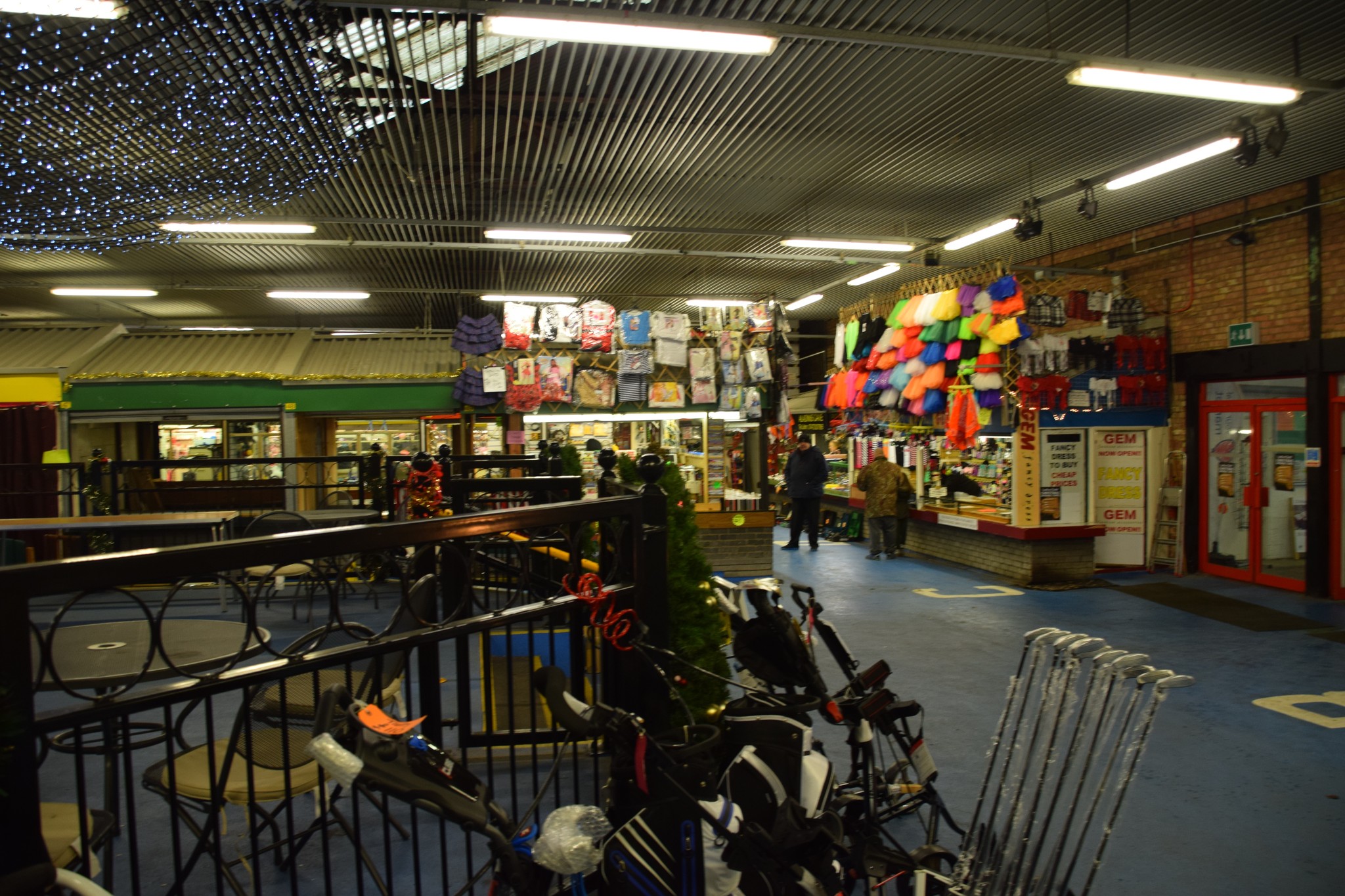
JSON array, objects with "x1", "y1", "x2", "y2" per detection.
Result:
[{"x1": 947, "y1": 626, "x2": 1196, "y2": 896}]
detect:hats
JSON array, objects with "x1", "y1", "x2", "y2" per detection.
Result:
[
  {"x1": 796, "y1": 434, "x2": 811, "y2": 444},
  {"x1": 873, "y1": 448, "x2": 883, "y2": 457}
]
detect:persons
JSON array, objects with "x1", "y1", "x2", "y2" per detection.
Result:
[
  {"x1": 772, "y1": 434, "x2": 980, "y2": 559},
  {"x1": 83, "y1": 449, "x2": 110, "y2": 513},
  {"x1": 237, "y1": 431, "x2": 581, "y2": 514}
]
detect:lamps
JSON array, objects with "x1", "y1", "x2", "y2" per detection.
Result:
[
  {"x1": 157, "y1": 220, "x2": 318, "y2": 234},
  {"x1": 266, "y1": 290, "x2": 371, "y2": 299},
  {"x1": 785, "y1": 293, "x2": 824, "y2": 311},
  {"x1": 1264, "y1": 113, "x2": 1289, "y2": 157},
  {"x1": 846, "y1": 265, "x2": 900, "y2": 285},
  {"x1": 780, "y1": 236, "x2": 916, "y2": 252},
  {"x1": 1013, "y1": 196, "x2": 1043, "y2": 242},
  {"x1": 943, "y1": 214, "x2": 1019, "y2": 251},
  {"x1": 1077, "y1": 187, "x2": 1098, "y2": 220},
  {"x1": 1102, "y1": 128, "x2": 1245, "y2": 191},
  {"x1": 482, "y1": 11, "x2": 780, "y2": 56},
  {"x1": 1234, "y1": 124, "x2": 1261, "y2": 169},
  {"x1": 1225, "y1": 230, "x2": 1256, "y2": 247},
  {"x1": 1062, "y1": 61, "x2": 1307, "y2": 107},
  {"x1": 923, "y1": 250, "x2": 941, "y2": 267},
  {"x1": 483, "y1": 226, "x2": 633, "y2": 242},
  {"x1": 685, "y1": 298, "x2": 753, "y2": 306}
]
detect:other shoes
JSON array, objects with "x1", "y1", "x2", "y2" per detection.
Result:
[
  {"x1": 781, "y1": 544, "x2": 798, "y2": 550},
  {"x1": 866, "y1": 554, "x2": 879, "y2": 560},
  {"x1": 810, "y1": 547, "x2": 817, "y2": 551},
  {"x1": 887, "y1": 553, "x2": 895, "y2": 559}
]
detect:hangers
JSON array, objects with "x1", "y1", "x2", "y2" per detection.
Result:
[
  {"x1": 849, "y1": 313, "x2": 857, "y2": 322},
  {"x1": 626, "y1": 301, "x2": 643, "y2": 313},
  {"x1": 463, "y1": 353, "x2": 505, "y2": 368},
  {"x1": 654, "y1": 303, "x2": 675, "y2": 317},
  {"x1": 458, "y1": 295, "x2": 498, "y2": 313}
]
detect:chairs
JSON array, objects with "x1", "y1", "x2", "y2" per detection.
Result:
[{"x1": 38, "y1": 490, "x2": 436, "y2": 896}]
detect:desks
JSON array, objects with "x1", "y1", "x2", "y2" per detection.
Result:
[
  {"x1": 0, "y1": 510, "x2": 239, "y2": 615},
  {"x1": 253, "y1": 508, "x2": 379, "y2": 610},
  {"x1": 30, "y1": 619, "x2": 271, "y2": 896}
]
